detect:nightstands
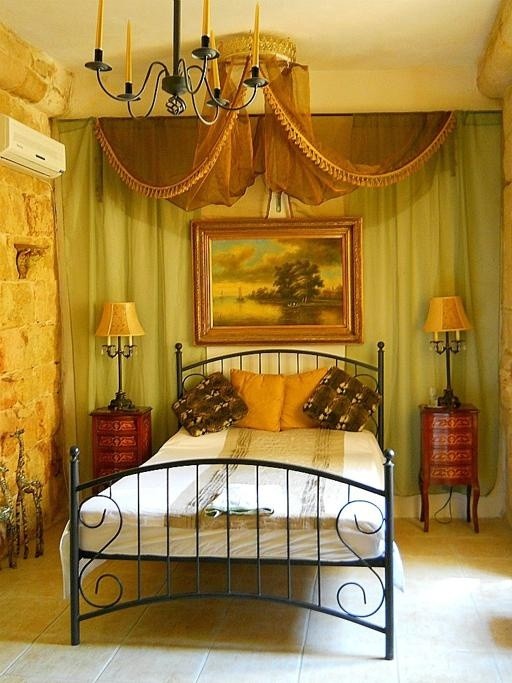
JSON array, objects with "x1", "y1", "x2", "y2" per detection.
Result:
[
  {"x1": 419, "y1": 406, "x2": 480, "y2": 533},
  {"x1": 90, "y1": 406, "x2": 152, "y2": 496}
]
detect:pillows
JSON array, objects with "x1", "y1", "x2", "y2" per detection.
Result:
[
  {"x1": 230, "y1": 369, "x2": 283, "y2": 432},
  {"x1": 280, "y1": 368, "x2": 327, "y2": 431},
  {"x1": 303, "y1": 366, "x2": 382, "y2": 431},
  {"x1": 171, "y1": 371, "x2": 248, "y2": 438}
]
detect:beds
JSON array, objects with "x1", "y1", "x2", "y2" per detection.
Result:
[{"x1": 71, "y1": 341, "x2": 394, "y2": 660}]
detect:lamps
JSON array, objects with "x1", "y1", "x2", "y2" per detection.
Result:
[
  {"x1": 423, "y1": 296, "x2": 474, "y2": 408},
  {"x1": 84, "y1": 0, "x2": 269, "y2": 125},
  {"x1": 95, "y1": 302, "x2": 145, "y2": 410}
]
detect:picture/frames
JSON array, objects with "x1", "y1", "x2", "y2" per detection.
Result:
[{"x1": 191, "y1": 218, "x2": 365, "y2": 344}]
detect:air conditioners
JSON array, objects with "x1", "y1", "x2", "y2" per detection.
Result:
[{"x1": 0, "y1": 114, "x2": 66, "y2": 179}]
